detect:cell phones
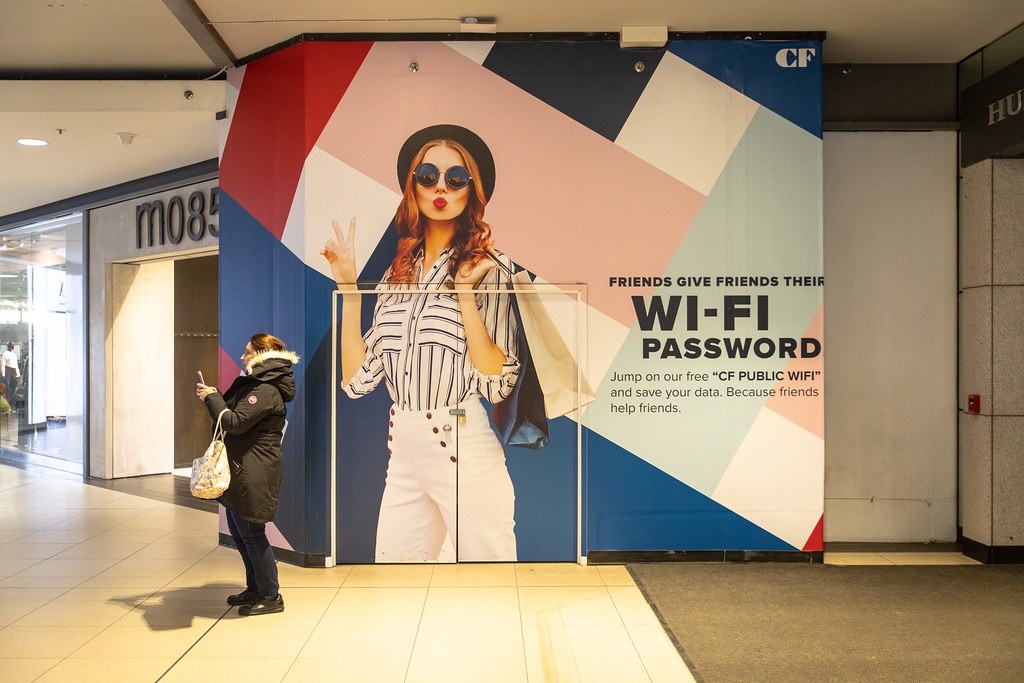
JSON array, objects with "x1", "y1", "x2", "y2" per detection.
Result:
[{"x1": 197, "y1": 371, "x2": 205, "y2": 385}]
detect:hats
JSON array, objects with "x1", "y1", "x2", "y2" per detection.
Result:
[
  {"x1": 7, "y1": 342, "x2": 13, "y2": 348},
  {"x1": 396, "y1": 124, "x2": 495, "y2": 206}
]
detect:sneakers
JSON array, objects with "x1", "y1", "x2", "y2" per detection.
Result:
[
  {"x1": 239, "y1": 594, "x2": 285, "y2": 616},
  {"x1": 227, "y1": 587, "x2": 258, "y2": 606}
]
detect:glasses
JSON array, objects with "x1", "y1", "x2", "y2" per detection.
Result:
[{"x1": 413, "y1": 163, "x2": 472, "y2": 189}]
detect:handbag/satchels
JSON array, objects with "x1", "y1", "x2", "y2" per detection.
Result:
[
  {"x1": 485, "y1": 248, "x2": 631, "y2": 420},
  {"x1": 190, "y1": 407, "x2": 232, "y2": 499},
  {"x1": 486, "y1": 251, "x2": 549, "y2": 449}
]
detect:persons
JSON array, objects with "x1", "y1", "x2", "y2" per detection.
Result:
[
  {"x1": 0, "y1": 341, "x2": 22, "y2": 413},
  {"x1": 319, "y1": 123, "x2": 524, "y2": 565},
  {"x1": 195, "y1": 332, "x2": 298, "y2": 618}
]
